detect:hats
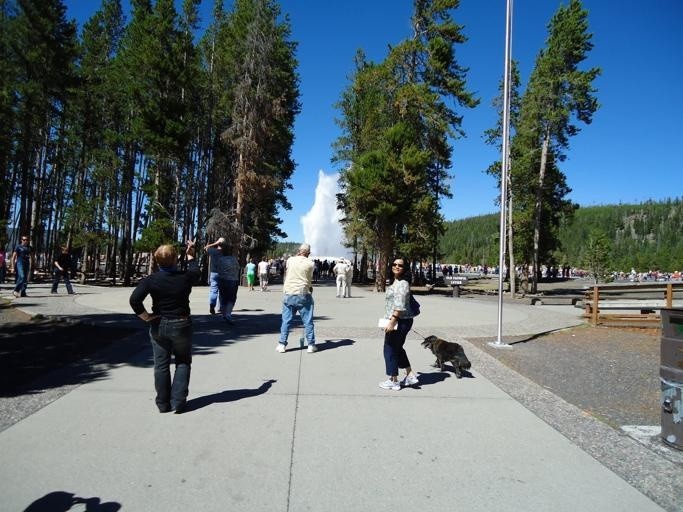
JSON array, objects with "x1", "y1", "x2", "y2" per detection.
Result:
[{"x1": 300, "y1": 243, "x2": 311, "y2": 252}]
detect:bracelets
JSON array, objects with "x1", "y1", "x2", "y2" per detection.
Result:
[
  {"x1": 391, "y1": 314, "x2": 399, "y2": 320},
  {"x1": 144, "y1": 315, "x2": 151, "y2": 322}
]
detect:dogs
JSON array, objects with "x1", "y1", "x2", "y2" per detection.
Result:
[{"x1": 421, "y1": 335, "x2": 471, "y2": 379}]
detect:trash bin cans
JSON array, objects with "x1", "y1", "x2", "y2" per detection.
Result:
[
  {"x1": 658, "y1": 306, "x2": 683, "y2": 451},
  {"x1": 436, "y1": 267, "x2": 439, "y2": 272},
  {"x1": 453, "y1": 284, "x2": 459, "y2": 297},
  {"x1": 242, "y1": 274, "x2": 249, "y2": 286},
  {"x1": 495, "y1": 269, "x2": 499, "y2": 275}
]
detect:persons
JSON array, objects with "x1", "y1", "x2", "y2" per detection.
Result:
[
  {"x1": 377, "y1": 257, "x2": 419, "y2": 391},
  {"x1": 213, "y1": 245, "x2": 240, "y2": 322},
  {"x1": 246, "y1": 256, "x2": 488, "y2": 298},
  {"x1": 50, "y1": 246, "x2": 76, "y2": 294},
  {"x1": 203, "y1": 238, "x2": 226, "y2": 315},
  {"x1": 0, "y1": 242, "x2": 8, "y2": 284},
  {"x1": 129, "y1": 236, "x2": 201, "y2": 413},
  {"x1": 277, "y1": 244, "x2": 318, "y2": 353},
  {"x1": 11, "y1": 235, "x2": 35, "y2": 298},
  {"x1": 561, "y1": 263, "x2": 683, "y2": 284}
]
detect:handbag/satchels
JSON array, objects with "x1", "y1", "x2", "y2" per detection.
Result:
[{"x1": 409, "y1": 294, "x2": 421, "y2": 318}]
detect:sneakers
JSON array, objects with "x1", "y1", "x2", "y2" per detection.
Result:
[
  {"x1": 276, "y1": 344, "x2": 286, "y2": 353},
  {"x1": 12, "y1": 291, "x2": 28, "y2": 298},
  {"x1": 307, "y1": 345, "x2": 319, "y2": 353},
  {"x1": 50, "y1": 291, "x2": 77, "y2": 295},
  {"x1": 176, "y1": 401, "x2": 192, "y2": 413},
  {"x1": 399, "y1": 373, "x2": 419, "y2": 387},
  {"x1": 210, "y1": 306, "x2": 233, "y2": 323},
  {"x1": 379, "y1": 380, "x2": 402, "y2": 391}
]
